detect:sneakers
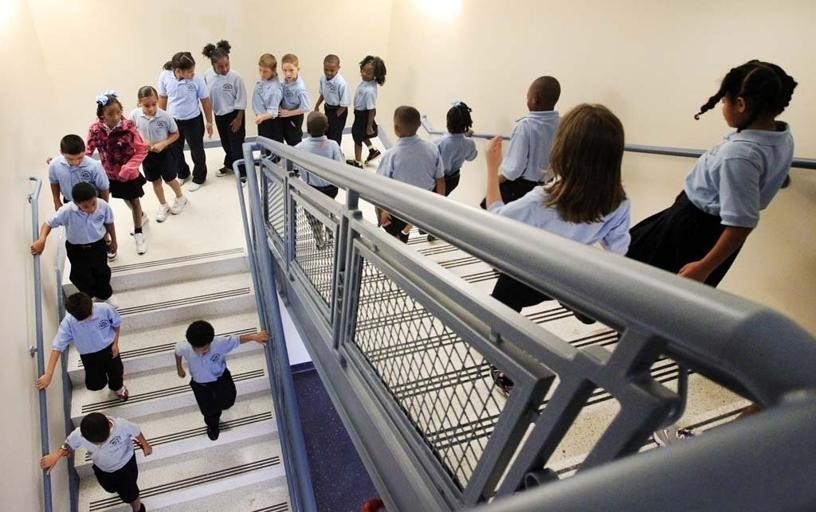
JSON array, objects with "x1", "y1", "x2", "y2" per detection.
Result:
[
  {"x1": 397, "y1": 226, "x2": 436, "y2": 245},
  {"x1": 490, "y1": 363, "x2": 515, "y2": 399},
  {"x1": 365, "y1": 148, "x2": 381, "y2": 165},
  {"x1": 155, "y1": 203, "x2": 171, "y2": 223},
  {"x1": 188, "y1": 179, "x2": 204, "y2": 192},
  {"x1": 129, "y1": 210, "x2": 149, "y2": 235},
  {"x1": 106, "y1": 242, "x2": 118, "y2": 262},
  {"x1": 653, "y1": 423, "x2": 700, "y2": 447},
  {"x1": 115, "y1": 385, "x2": 128, "y2": 400},
  {"x1": 175, "y1": 176, "x2": 186, "y2": 187},
  {"x1": 134, "y1": 233, "x2": 146, "y2": 254},
  {"x1": 132, "y1": 502, "x2": 146, "y2": 512},
  {"x1": 95, "y1": 295, "x2": 118, "y2": 309},
  {"x1": 203, "y1": 422, "x2": 222, "y2": 440},
  {"x1": 215, "y1": 165, "x2": 235, "y2": 178},
  {"x1": 346, "y1": 160, "x2": 364, "y2": 170},
  {"x1": 238, "y1": 175, "x2": 247, "y2": 187},
  {"x1": 171, "y1": 196, "x2": 189, "y2": 216}
]
